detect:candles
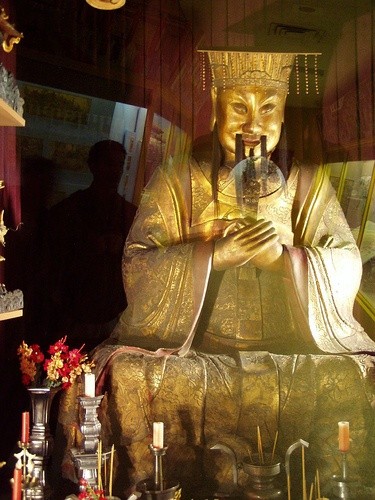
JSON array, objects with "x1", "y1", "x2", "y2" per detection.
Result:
[
  {"x1": 12, "y1": 459, "x2": 22, "y2": 500},
  {"x1": 85, "y1": 371, "x2": 96, "y2": 398},
  {"x1": 337, "y1": 420, "x2": 350, "y2": 450},
  {"x1": 21, "y1": 412, "x2": 30, "y2": 443},
  {"x1": 152, "y1": 421, "x2": 164, "y2": 448}
]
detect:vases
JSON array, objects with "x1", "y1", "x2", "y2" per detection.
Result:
[{"x1": 31, "y1": 390, "x2": 58, "y2": 426}]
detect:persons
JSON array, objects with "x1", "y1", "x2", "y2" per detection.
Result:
[
  {"x1": 89, "y1": 50, "x2": 375, "y2": 500},
  {"x1": 37, "y1": 140, "x2": 138, "y2": 322}
]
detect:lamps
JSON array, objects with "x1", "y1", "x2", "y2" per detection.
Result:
[{"x1": 84, "y1": 0, "x2": 127, "y2": 12}]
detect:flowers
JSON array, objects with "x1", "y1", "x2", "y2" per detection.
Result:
[
  {"x1": 78, "y1": 476, "x2": 108, "y2": 500},
  {"x1": 17, "y1": 332, "x2": 93, "y2": 392}
]
